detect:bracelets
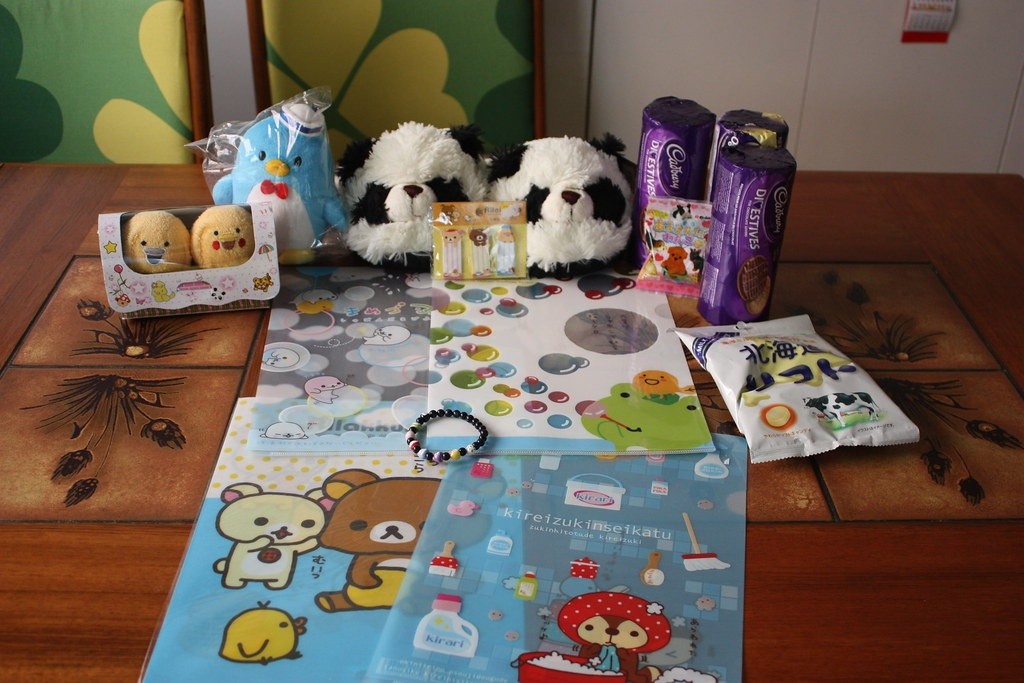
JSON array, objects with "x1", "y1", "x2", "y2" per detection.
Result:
[{"x1": 406, "y1": 409, "x2": 488, "y2": 463}]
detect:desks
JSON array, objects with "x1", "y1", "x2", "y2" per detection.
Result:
[{"x1": 1, "y1": 164, "x2": 1024, "y2": 683}]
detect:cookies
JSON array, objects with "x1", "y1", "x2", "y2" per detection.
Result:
[{"x1": 737, "y1": 255, "x2": 771, "y2": 315}]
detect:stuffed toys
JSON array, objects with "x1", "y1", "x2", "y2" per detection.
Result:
[
  {"x1": 334, "y1": 121, "x2": 489, "y2": 277},
  {"x1": 190, "y1": 205, "x2": 255, "y2": 269},
  {"x1": 212, "y1": 104, "x2": 350, "y2": 265},
  {"x1": 121, "y1": 211, "x2": 192, "y2": 274},
  {"x1": 486, "y1": 133, "x2": 635, "y2": 279}
]
